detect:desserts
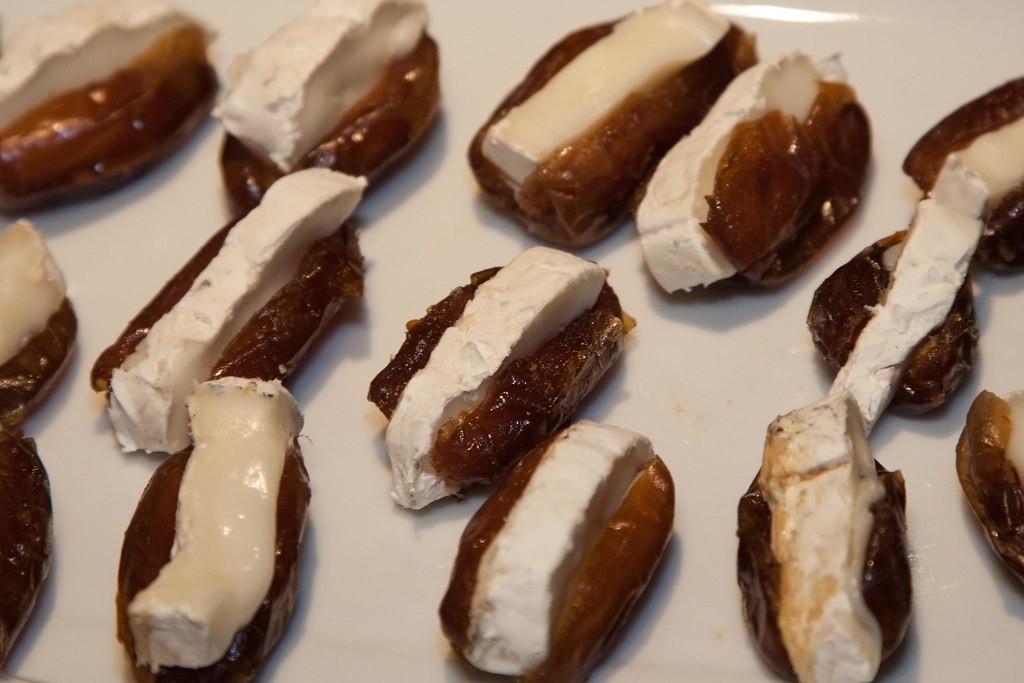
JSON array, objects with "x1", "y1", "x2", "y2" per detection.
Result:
[{"x1": 0, "y1": 0, "x2": 1024, "y2": 683}]
